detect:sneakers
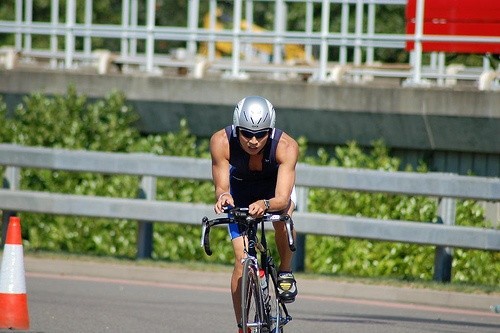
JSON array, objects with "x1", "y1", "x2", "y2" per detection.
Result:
[
  {"x1": 239, "y1": 327, "x2": 251, "y2": 333},
  {"x1": 277, "y1": 270, "x2": 297, "y2": 303}
]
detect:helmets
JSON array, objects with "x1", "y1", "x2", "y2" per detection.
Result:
[{"x1": 232, "y1": 96, "x2": 276, "y2": 139}]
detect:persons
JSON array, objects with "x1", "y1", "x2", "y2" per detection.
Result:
[{"x1": 209, "y1": 95, "x2": 298, "y2": 333}]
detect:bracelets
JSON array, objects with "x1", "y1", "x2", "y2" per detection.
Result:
[{"x1": 218, "y1": 192, "x2": 231, "y2": 200}]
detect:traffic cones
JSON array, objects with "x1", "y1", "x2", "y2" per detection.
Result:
[{"x1": 0, "y1": 216, "x2": 29, "y2": 331}]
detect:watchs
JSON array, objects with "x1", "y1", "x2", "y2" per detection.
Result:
[{"x1": 263, "y1": 199, "x2": 270, "y2": 215}]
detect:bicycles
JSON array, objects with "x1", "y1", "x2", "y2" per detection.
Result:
[{"x1": 201, "y1": 202, "x2": 297, "y2": 333}]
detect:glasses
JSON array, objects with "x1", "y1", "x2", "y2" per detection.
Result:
[{"x1": 239, "y1": 127, "x2": 269, "y2": 138}]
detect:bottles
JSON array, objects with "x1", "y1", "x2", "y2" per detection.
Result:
[{"x1": 259, "y1": 267, "x2": 269, "y2": 303}]
